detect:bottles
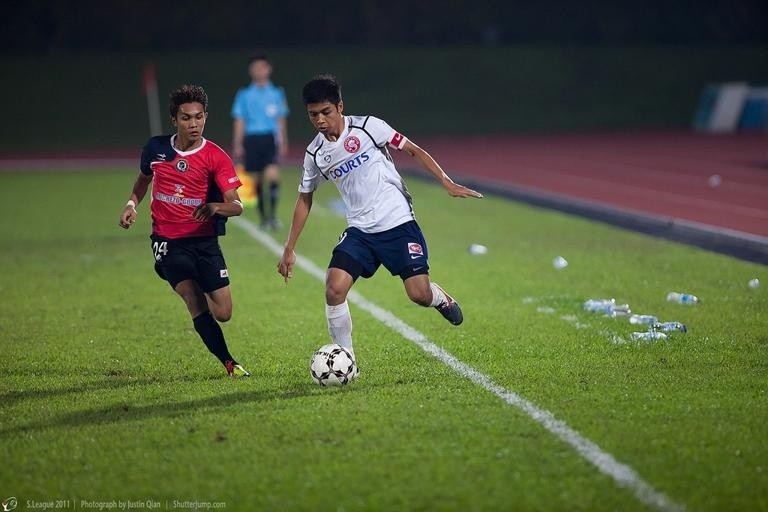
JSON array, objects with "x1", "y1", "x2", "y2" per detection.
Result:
[
  {"x1": 584, "y1": 297, "x2": 687, "y2": 340},
  {"x1": 668, "y1": 292, "x2": 700, "y2": 306}
]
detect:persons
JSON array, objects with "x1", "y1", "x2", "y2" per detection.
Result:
[
  {"x1": 276, "y1": 74, "x2": 483, "y2": 379},
  {"x1": 230, "y1": 59, "x2": 289, "y2": 231},
  {"x1": 119, "y1": 83, "x2": 252, "y2": 380}
]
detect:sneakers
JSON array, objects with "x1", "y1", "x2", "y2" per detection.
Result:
[
  {"x1": 223, "y1": 359, "x2": 248, "y2": 375},
  {"x1": 429, "y1": 281, "x2": 463, "y2": 326}
]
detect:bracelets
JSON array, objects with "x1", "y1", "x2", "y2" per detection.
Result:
[{"x1": 127, "y1": 200, "x2": 136, "y2": 208}]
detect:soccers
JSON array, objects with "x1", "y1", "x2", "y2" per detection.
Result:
[{"x1": 310, "y1": 344, "x2": 355, "y2": 386}]
